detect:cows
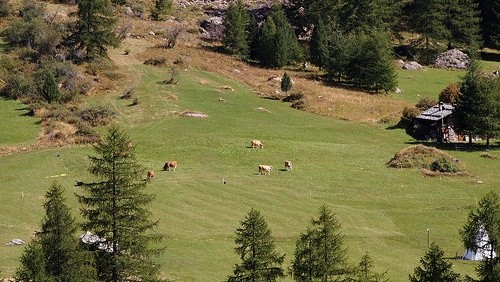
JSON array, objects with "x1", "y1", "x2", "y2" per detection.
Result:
[
  {"x1": 258, "y1": 164, "x2": 273, "y2": 176},
  {"x1": 162, "y1": 161, "x2": 177, "y2": 172},
  {"x1": 250, "y1": 140, "x2": 265, "y2": 150},
  {"x1": 146, "y1": 170, "x2": 155, "y2": 182},
  {"x1": 284, "y1": 160, "x2": 292, "y2": 171}
]
